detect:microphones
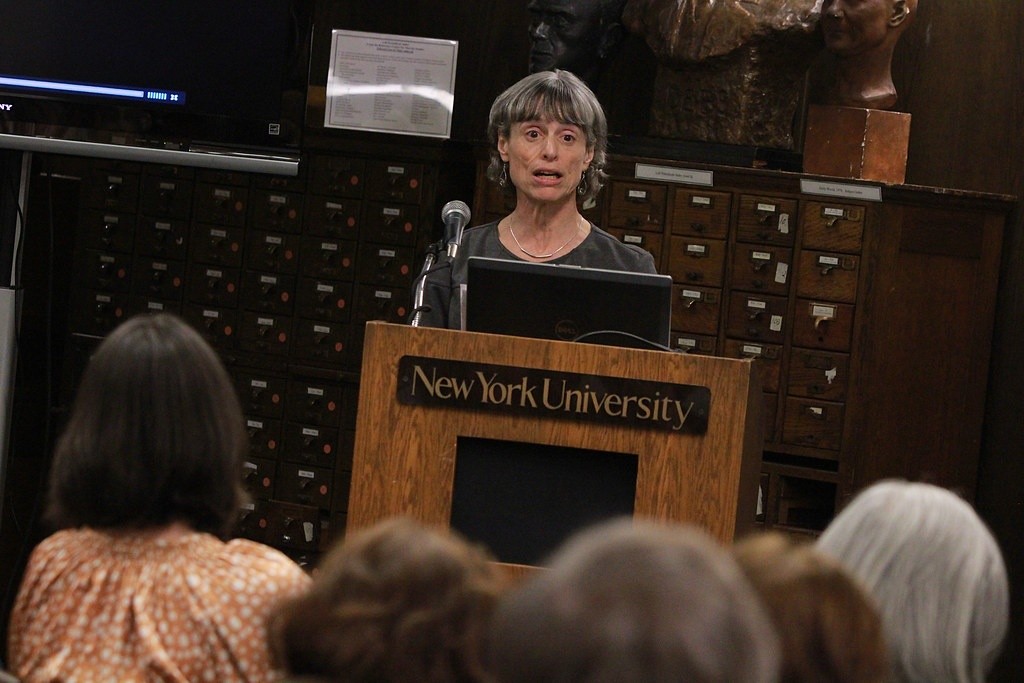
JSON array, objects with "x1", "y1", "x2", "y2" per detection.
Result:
[{"x1": 442, "y1": 200, "x2": 471, "y2": 268}]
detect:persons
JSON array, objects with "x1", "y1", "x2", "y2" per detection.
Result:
[
  {"x1": 408, "y1": 68, "x2": 659, "y2": 331},
  {"x1": 7, "y1": 314, "x2": 319, "y2": 683},
  {"x1": 811, "y1": 0, "x2": 920, "y2": 110},
  {"x1": 263, "y1": 514, "x2": 514, "y2": 682},
  {"x1": 814, "y1": 480, "x2": 1010, "y2": 683},
  {"x1": 728, "y1": 529, "x2": 914, "y2": 682},
  {"x1": 474, "y1": 517, "x2": 784, "y2": 683},
  {"x1": 526, "y1": 0, "x2": 643, "y2": 133}
]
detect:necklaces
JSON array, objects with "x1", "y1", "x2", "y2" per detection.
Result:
[{"x1": 508, "y1": 214, "x2": 584, "y2": 259}]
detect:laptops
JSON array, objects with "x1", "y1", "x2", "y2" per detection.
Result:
[{"x1": 465, "y1": 256, "x2": 673, "y2": 350}]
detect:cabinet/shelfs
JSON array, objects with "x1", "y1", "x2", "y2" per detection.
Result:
[{"x1": 1, "y1": 124, "x2": 1023, "y2": 576}]
